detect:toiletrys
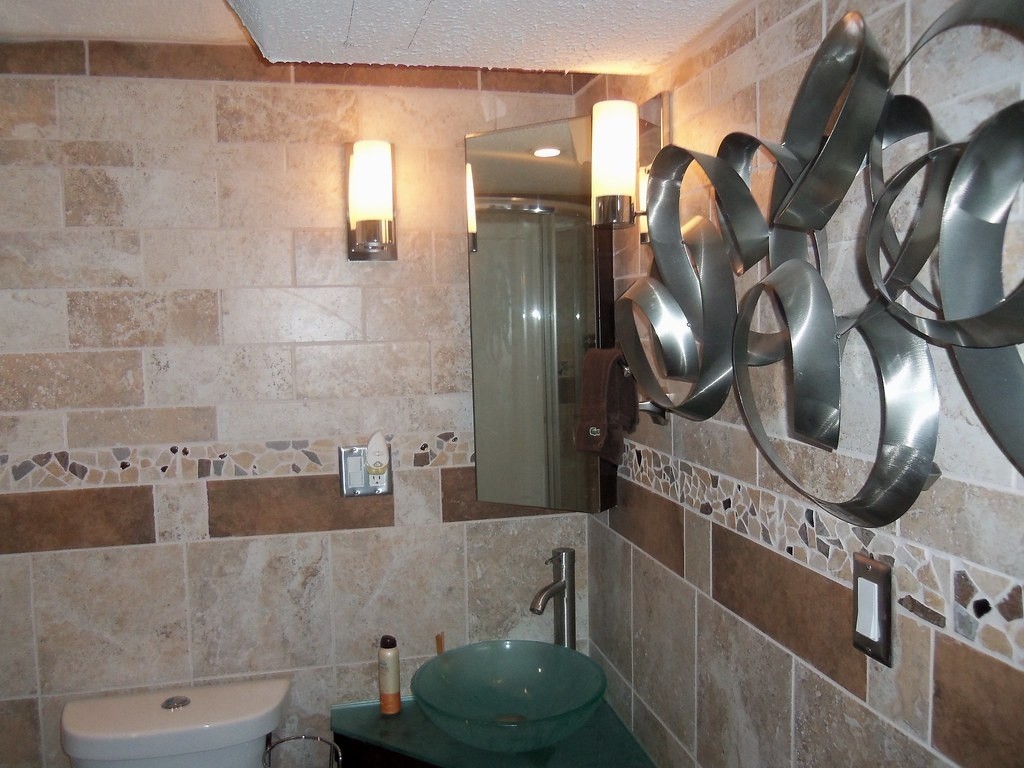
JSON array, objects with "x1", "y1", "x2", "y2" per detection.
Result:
[{"x1": 378, "y1": 634, "x2": 401, "y2": 715}]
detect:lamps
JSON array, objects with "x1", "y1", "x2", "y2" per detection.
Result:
[
  {"x1": 588, "y1": 89, "x2": 673, "y2": 230},
  {"x1": 346, "y1": 138, "x2": 401, "y2": 262}
]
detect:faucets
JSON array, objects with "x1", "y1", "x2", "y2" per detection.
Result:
[{"x1": 528, "y1": 546, "x2": 577, "y2": 650}]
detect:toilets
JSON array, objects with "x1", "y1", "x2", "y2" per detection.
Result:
[{"x1": 62, "y1": 679, "x2": 293, "y2": 767}]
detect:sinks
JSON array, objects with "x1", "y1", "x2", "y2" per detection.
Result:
[{"x1": 410, "y1": 640, "x2": 607, "y2": 753}]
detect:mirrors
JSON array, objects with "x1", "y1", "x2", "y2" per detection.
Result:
[{"x1": 462, "y1": 115, "x2": 620, "y2": 517}]
